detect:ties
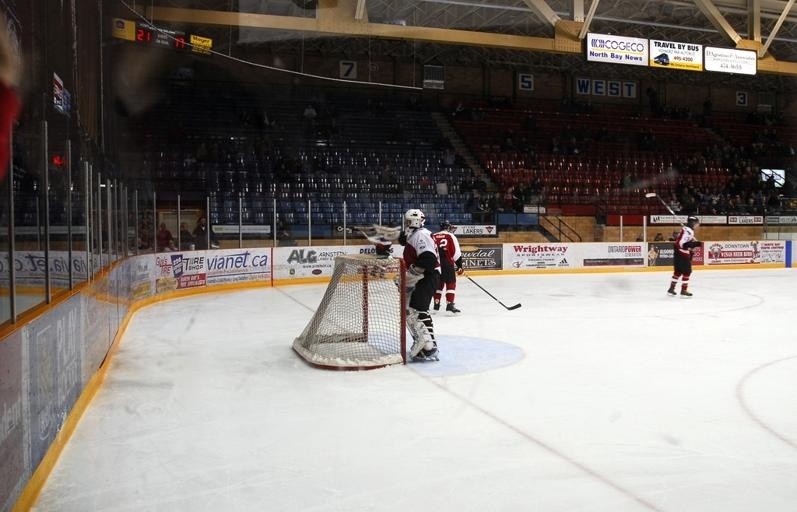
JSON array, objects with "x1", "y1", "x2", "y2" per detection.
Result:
[{"x1": 761, "y1": 168, "x2": 786, "y2": 188}]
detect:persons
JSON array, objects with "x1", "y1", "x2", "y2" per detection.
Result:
[
  {"x1": 635, "y1": 233, "x2": 643, "y2": 242},
  {"x1": 675, "y1": 174, "x2": 793, "y2": 215},
  {"x1": 368, "y1": 234, "x2": 393, "y2": 277},
  {"x1": 430, "y1": 220, "x2": 464, "y2": 314},
  {"x1": 391, "y1": 208, "x2": 442, "y2": 362},
  {"x1": 621, "y1": 172, "x2": 632, "y2": 186},
  {"x1": 655, "y1": 233, "x2": 664, "y2": 239},
  {"x1": 667, "y1": 216, "x2": 702, "y2": 297},
  {"x1": 647, "y1": 243, "x2": 659, "y2": 265},
  {"x1": 127, "y1": 208, "x2": 216, "y2": 256},
  {"x1": 669, "y1": 230, "x2": 678, "y2": 241},
  {"x1": 277, "y1": 226, "x2": 292, "y2": 246},
  {"x1": 460, "y1": 174, "x2": 546, "y2": 225}
]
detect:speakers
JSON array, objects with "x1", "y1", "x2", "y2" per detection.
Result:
[
  {"x1": 667, "y1": 288, "x2": 677, "y2": 295},
  {"x1": 680, "y1": 290, "x2": 693, "y2": 296},
  {"x1": 433, "y1": 302, "x2": 440, "y2": 311},
  {"x1": 446, "y1": 303, "x2": 461, "y2": 313}
]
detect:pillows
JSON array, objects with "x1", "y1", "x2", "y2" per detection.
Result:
[
  {"x1": 405, "y1": 209, "x2": 426, "y2": 228},
  {"x1": 439, "y1": 219, "x2": 451, "y2": 227},
  {"x1": 687, "y1": 216, "x2": 699, "y2": 224}
]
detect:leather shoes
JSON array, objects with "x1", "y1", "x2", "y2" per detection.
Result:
[{"x1": 456, "y1": 268, "x2": 465, "y2": 275}]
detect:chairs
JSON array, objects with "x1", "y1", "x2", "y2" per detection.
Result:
[{"x1": 202, "y1": 100, "x2": 797, "y2": 227}]
